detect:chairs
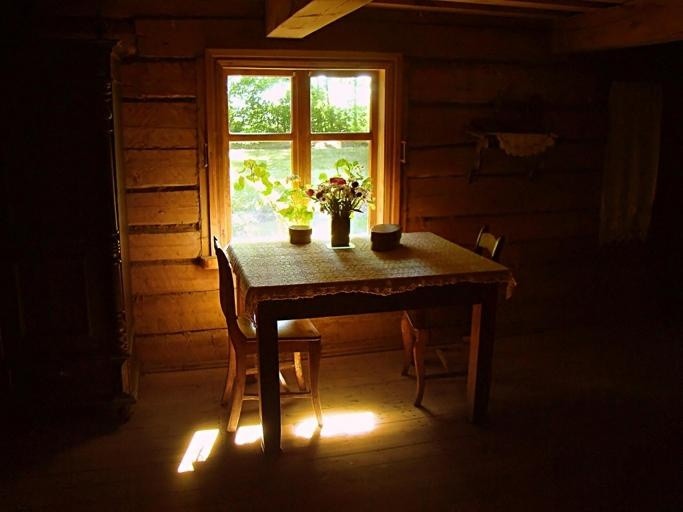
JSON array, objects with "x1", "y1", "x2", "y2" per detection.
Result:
[
  {"x1": 401, "y1": 223, "x2": 505, "y2": 408},
  {"x1": 213, "y1": 236, "x2": 324, "y2": 432}
]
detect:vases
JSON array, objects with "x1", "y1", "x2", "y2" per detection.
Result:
[{"x1": 330, "y1": 214, "x2": 350, "y2": 248}]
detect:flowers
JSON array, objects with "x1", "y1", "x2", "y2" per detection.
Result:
[{"x1": 305, "y1": 178, "x2": 370, "y2": 217}]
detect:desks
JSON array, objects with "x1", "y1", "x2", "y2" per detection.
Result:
[{"x1": 225, "y1": 230, "x2": 519, "y2": 455}]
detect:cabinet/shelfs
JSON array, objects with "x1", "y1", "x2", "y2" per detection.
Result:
[{"x1": 1, "y1": 39, "x2": 141, "y2": 436}]
